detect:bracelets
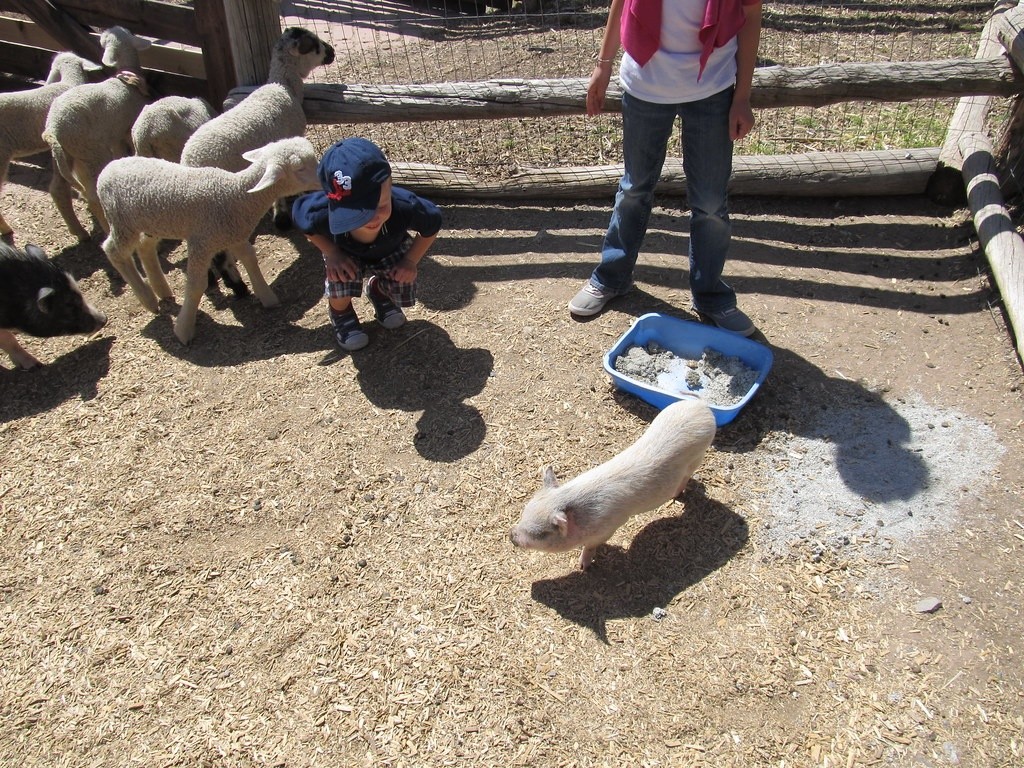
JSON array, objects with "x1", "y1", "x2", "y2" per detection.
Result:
[{"x1": 595, "y1": 58, "x2": 615, "y2": 62}]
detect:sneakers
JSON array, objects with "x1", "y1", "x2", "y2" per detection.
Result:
[
  {"x1": 366, "y1": 275, "x2": 405, "y2": 329},
  {"x1": 568, "y1": 284, "x2": 618, "y2": 316},
  {"x1": 693, "y1": 307, "x2": 756, "y2": 337},
  {"x1": 326, "y1": 300, "x2": 369, "y2": 350}
]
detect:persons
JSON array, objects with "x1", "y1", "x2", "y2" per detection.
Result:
[
  {"x1": 566, "y1": 0, "x2": 764, "y2": 340},
  {"x1": 292, "y1": 136, "x2": 443, "y2": 352}
]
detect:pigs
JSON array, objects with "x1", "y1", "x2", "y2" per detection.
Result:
[
  {"x1": 0, "y1": 239, "x2": 107, "y2": 372},
  {"x1": 508, "y1": 390, "x2": 716, "y2": 570}
]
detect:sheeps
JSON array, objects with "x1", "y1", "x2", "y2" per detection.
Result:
[{"x1": 0, "y1": 24, "x2": 335, "y2": 344}]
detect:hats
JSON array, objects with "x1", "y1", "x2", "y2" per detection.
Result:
[{"x1": 317, "y1": 137, "x2": 392, "y2": 235}]
